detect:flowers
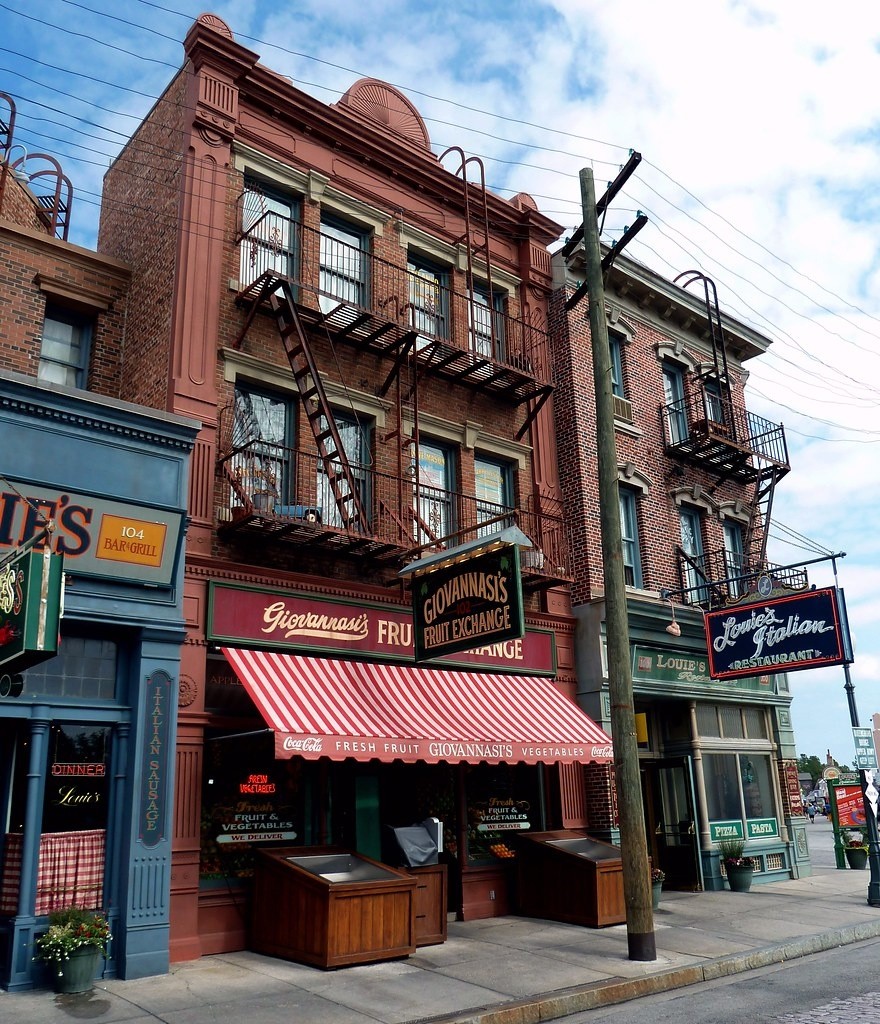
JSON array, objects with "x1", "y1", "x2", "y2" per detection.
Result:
[
  {"x1": 650, "y1": 868, "x2": 666, "y2": 882},
  {"x1": 718, "y1": 837, "x2": 756, "y2": 866},
  {"x1": 843, "y1": 840, "x2": 869, "y2": 856},
  {"x1": 24, "y1": 904, "x2": 114, "y2": 976}
]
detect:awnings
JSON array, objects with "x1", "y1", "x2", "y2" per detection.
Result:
[{"x1": 217, "y1": 644, "x2": 616, "y2": 768}]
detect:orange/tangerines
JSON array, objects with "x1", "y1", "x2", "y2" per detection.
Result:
[{"x1": 490, "y1": 844, "x2": 514, "y2": 858}]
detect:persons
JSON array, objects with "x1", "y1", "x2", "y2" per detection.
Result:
[{"x1": 803, "y1": 802, "x2": 818, "y2": 824}]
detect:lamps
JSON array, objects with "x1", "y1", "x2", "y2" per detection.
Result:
[
  {"x1": 659, "y1": 597, "x2": 682, "y2": 636},
  {"x1": 0, "y1": 144, "x2": 30, "y2": 183}
]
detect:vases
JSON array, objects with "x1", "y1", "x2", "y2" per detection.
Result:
[
  {"x1": 52, "y1": 946, "x2": 98, "y2": 993},
  {"x1": 652, "y1": 881, "x2": 661, "y2": 910},
  {"x1": 725, "y1": 865, "x2": 754, "y2": 893},
  {"x1": 845, "y1": 849, "x2": 867, "y2": 869}
]
